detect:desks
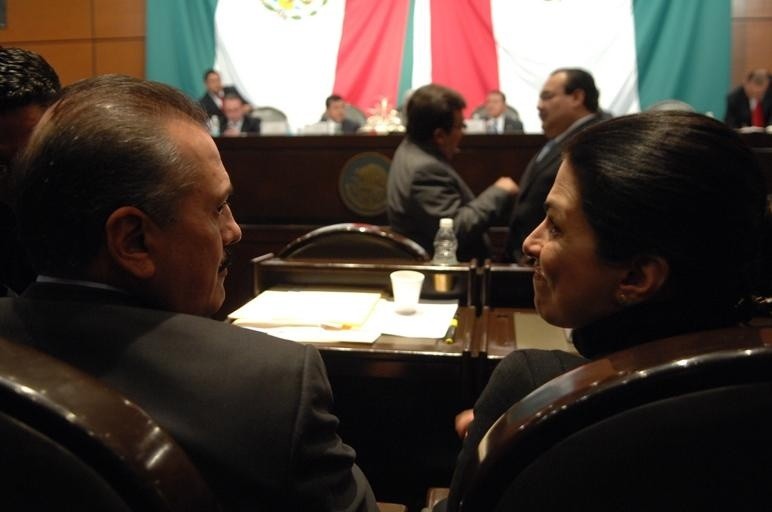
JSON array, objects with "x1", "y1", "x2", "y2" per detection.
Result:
[
  {"x1": 222, "y1": 253, "x2": 480, "y2": 365},
  {"x1": 480, "y1": 258, "x2": 582, "y2": 361}
]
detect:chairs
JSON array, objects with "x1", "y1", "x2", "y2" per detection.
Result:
[
  {"x1": 274, "y1": 223, "x2": 429, "y2": 262},
  {"x1": 0, "y1": 337, "x2": 406, "y2": 512},
  {"x1": 425, "y1": 322, "x2": 772, "y2": 511}
]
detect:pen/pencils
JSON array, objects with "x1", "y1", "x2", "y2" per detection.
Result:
[{"x1": 444, "y1": 313, "x2": 459, "y2": 344}]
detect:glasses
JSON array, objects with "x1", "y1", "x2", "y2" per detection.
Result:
[{"x1": 452, "y1": 121, "x2": 466, "y2": 133}]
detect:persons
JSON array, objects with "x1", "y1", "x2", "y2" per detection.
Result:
[
  {"x1": 219, "y1": 91, "x2": 260, "y2": 137},
  {"x1": 449, "y1": 108, "x2": 771, "y2": 511},
  {"x1": 386, "y1": 83, "x2": 520, "y2": 262},
  {"x1": 321, "y1": 95, "x2": 360, "y2": 134},
  {"x1": 197, "y1": 68, "x2": 249, "y2": 118},
  {"x1": 0, "y1": 69, "x2": 380, "y2": 510},
  {"x1": 475, "y1": 89, "x2": 524, "y2": 132},
  {"x1": 0, "y1": 45, "x2": 63, "y2": 162},
  {"x1": 504, "y1": 67, "x2": 612, "y2": 266},
  {"x1": 723, "y1": 66, "x2": 772, "y2": 129}
]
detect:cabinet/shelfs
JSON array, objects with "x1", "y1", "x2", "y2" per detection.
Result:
[{"x1": 212, "y1": 133, "x2": 550, "y2": 224}]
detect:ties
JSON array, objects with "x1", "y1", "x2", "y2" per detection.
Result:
[
  {"x1": 219, "y1": 96, "x2": 225, "y2": 112},
  {"x1": 493, "y1": 119, "x2": 498, "y2": 134},
  {"x1": 752, "y1": 101, "x2": 764, "y2": 127},
  {"x1": 537, "y1": 139, "x2": 556, "y2": 163}
]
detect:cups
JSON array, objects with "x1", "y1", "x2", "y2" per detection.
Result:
[{"x1": 390, "y1": 271, "x2": 424, "y2": 313}]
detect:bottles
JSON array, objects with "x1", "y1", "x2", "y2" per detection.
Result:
[
  {"x1": 432, "y1": 218, "x2": 459, "y2": 263},
  {"x1": 211, "y1": 115, "x2": 220, "y2": 137}
]
